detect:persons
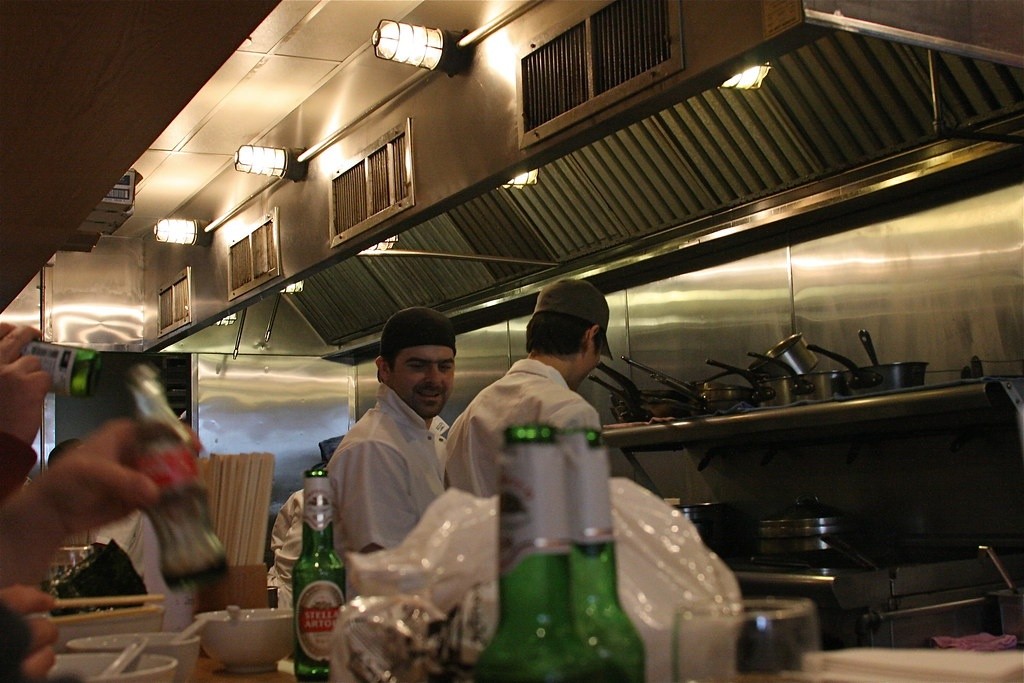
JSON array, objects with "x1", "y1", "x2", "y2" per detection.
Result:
[
  {"x1": 328, "y1": 305, "x2": 456, "y2": 560},
  {"x1": 1, "y1": 321, "x2": 204, "y2": 683},
  {"x1": 272, "y1": 490, "x2": 306, "y2": 609},
  {"x1": 445, "y1": 278, "x2": 613, "y2": 495}
]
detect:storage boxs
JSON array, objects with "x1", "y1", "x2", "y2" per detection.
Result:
[{"x1": 59, "y1": 168, "x2": 143, "y2": 253}]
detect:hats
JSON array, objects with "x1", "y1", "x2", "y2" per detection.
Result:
[
  {"x1": 379, "y1": 306, "x2": 458, "y2": 359},
  {"x1": 533, "y1": 279, "x2": 614, "y2": 363}
]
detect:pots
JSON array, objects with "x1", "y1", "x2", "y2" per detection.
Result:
[
  {"x1": 758, "y1": 493, "x2": 844, "y2": 552},
  {"x1": 587, "y1": 329, "x2": 930, "y2": 423},
  {"x1": 673, "y1": 502, "x2": 735, "y2": 558}
]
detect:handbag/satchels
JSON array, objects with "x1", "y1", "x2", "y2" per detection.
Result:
[{"x1": 343, "y1": 477, "x2": 746, "y2": 683}]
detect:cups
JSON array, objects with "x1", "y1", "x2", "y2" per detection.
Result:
[
  {"x1": 673, "y1": 598, "x2": 820, "y2": 683},
  {"x1": 196, "y1": 564, "x2": 268, "y2": 657}
]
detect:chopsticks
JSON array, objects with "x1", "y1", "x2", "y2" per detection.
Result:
[
  {"x1": 195, "y1": 452, "x2": 275, "y2": 564},
  {"x1": 47, "y1": 593, "x2": 165, "y2": 623}
]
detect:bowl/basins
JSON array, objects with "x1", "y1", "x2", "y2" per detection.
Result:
[
  {"x1": 66, "y1": 631, "x2": 201, "y2": 683},
  {"x1": 195, "y1": 609, "x2": 294, "y2": 672},
  {"x1": 52, "y1": 605, "x2": 163, "y2": 651},
  {"x1": 46, "y1": 653, "x2": 177, "y2": 683}
]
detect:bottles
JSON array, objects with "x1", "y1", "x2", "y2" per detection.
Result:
[
  {"x1": 475, "y1": 423, "x2": 645, "y2": 683},
  {"x1": 293, "y1": 469, "x2": 346, "y2": 683},
  {"x1": 19, "y1": 340, "x2": 100, "y2": 398},
  {"x1": 121, "y1": 363, "x2": 230, "y2": 590}
]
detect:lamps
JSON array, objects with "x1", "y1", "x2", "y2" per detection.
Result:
[
  {"x1": 371, "y1": 18, "x2": 473, "y2": 79},
  {"x1": 215, "y1": 312, "x2": 238, "y2": 325},
  {"x1": 154, "y1": 218, "x2": 211, "y2": 246},
  {"x1": 234, "y1": 143, "x2": 308, "y2": 182},
  {"x1": 361, "y1": 235, "x2": 398, "y2": 251},
  {"x1": 496, "y1": 168, "x2": 539, "y2": 190},
  {"x1": 722, "y1": 61, "x2": 774, "y2": 90},
  {"x1": 280, "y1": 281, "x2": 304, "y2": 293}
]
